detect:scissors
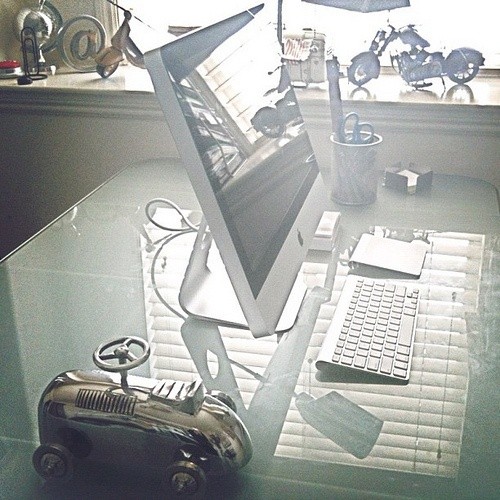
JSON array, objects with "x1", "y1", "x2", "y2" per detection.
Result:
[{"x1": 340, "y1": 112, "x2": 374, "y2": 145}]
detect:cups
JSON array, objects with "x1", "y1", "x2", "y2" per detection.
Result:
[{"x1": 329, "y1": 131, "x2": 383, "y2": 206}]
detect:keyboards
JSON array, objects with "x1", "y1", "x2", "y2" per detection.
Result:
[{"x1": 315, "y1": 273, "x2": 422, "y2": 384}]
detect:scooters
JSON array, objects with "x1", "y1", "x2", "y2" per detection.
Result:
[{"x1": 97, "y1": 6, "x2": 149, "y2": 80}]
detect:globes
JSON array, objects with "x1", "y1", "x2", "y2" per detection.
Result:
[{"x1": 12, "y1": 1, "x2": 63, "y2": 75}]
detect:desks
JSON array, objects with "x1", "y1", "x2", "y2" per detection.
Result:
[{"x1": 0, "y1": 154, "x2": 500, "y2": 498}]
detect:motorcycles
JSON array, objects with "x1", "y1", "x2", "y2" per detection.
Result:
[{"x1": 347, "y1": 8, "x2": 485, "y2": 89}]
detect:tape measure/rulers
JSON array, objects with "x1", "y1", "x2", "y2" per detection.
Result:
[{"x1": 326, "y1": 59, "x2": 345, "y2": 143}]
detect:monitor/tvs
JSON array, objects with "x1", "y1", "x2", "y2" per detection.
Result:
[{"x1": 142, "y1": 2, "x2": 330, "y2": 338}]
